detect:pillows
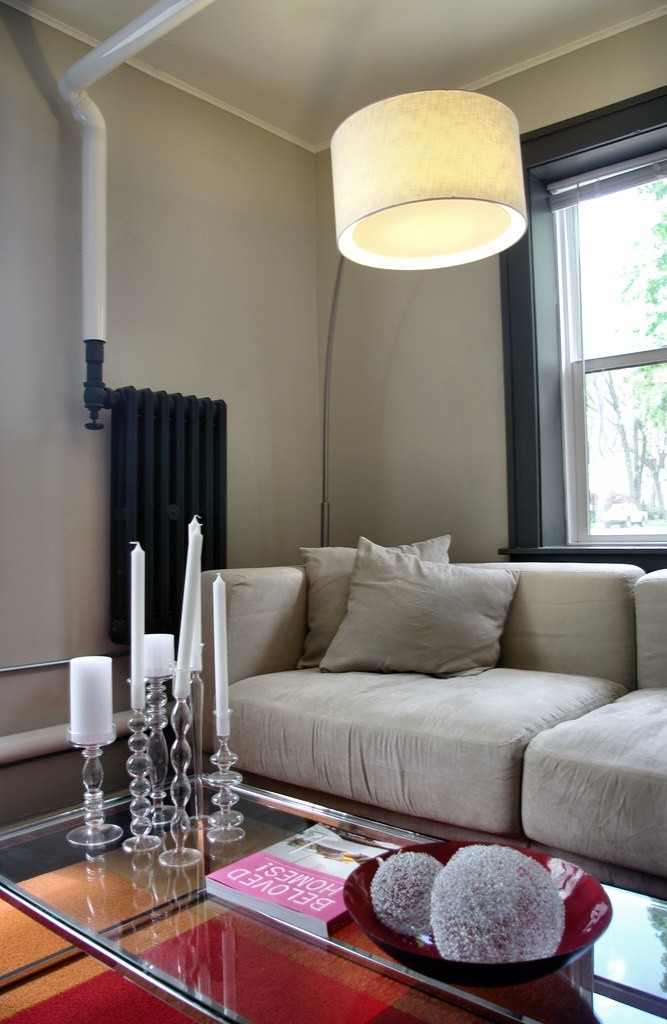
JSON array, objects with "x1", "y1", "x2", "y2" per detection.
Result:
[
  {"x1": 321, "y1": 534, "x2": 522, "y2": 678},
  {"x1": 296, "y1": 535, "x2": 456, "y2": 668}
]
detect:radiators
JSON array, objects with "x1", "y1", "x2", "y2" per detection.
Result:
[{"x1": 104, "y1": 385, "x2": 227, "y2": 645}]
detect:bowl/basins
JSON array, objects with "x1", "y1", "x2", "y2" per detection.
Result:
[{"x1": 343, "y1": 841, "x2": 613, "y2": 987}]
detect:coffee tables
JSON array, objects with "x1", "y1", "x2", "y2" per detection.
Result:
[{"x1": 0, "y1": 774, "x2": 667, "y2": 1022}]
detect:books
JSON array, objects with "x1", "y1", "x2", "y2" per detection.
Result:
[{"x1": 205, "y1": 814, "x2": 448, "y2": 940}]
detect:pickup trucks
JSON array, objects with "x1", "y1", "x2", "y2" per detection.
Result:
[{"x1": 605, "y1": 504, "x2": 647, "y2": 528}]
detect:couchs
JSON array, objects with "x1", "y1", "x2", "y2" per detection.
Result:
[{"x1": 191, "y1": 560, "x2": 667, "y2": 876}]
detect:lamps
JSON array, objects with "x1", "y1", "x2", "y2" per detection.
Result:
[{"x1": 321, "y1": 89, "x2": 529, "y2": 546}]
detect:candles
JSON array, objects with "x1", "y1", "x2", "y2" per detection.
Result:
[
  {"x1": 131, "y1": 541, "x2": 147, "y2": 708},
  {"x1": 211, "y1": 573, "x2": 233, "y2": 736},
  {"x1": 71, "y1": 656, "x2": 112, "y2": 743},
  {"x1": 175, "y1": 524, "x2": 201, "y2": 694},
  {"x1": 189, "y1": 511, "x2": 203, "y2": 671}
]
